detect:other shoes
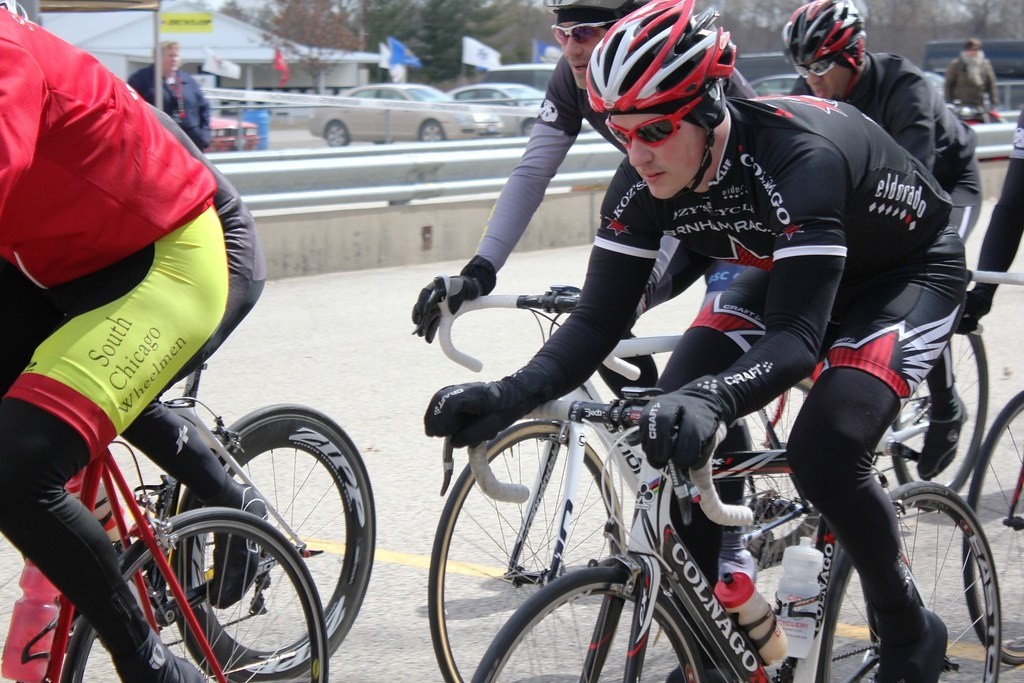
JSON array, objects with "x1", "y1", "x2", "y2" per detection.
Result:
[{"x1": 716, "y1": 527, "x2": 756, "y2": 585}]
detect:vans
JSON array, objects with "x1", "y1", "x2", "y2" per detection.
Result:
[{"x1": 482, "y1": 64, "x2": 556, "y2": 92}]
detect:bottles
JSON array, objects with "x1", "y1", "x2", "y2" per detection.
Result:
[
  {"x1": 64, "y1": 469, "x2": 130, "y2": 543},
  {"x1": 0, "y1": 557, "x2": 61, "y2": 683},
  {"x1": 772, "y1": 535, "x2": 824, "y2": 659},
  {"x1": 714, "y1": 571, "x2": 788, "y2": 665}
]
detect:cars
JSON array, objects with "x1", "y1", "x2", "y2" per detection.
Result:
[
  {"x1": 308, "y1": 83, "x2": 593, "y2": 147},
  {"x1": 749, "y1": 75, "x2": 800, "y2": 96},
  {"x1": 202, "y1": 117, "x2": 260, "y2": 152}
]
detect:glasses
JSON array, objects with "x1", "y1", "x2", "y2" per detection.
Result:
[
  {"x1": 795, "y1": 39, "x2": 858, "y2": 78},
  {"x1": 551, "y1": 18, "x2": 621, "y2": 45},
  {"x1": 604, "y1": 79, "x2": 719, "y2": 148}
]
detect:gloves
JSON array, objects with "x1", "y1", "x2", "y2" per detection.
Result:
[
  {"x1": 639, "y1": 374, "x2": 741, "y2": 468},
  {"x1": 412, "y1": 275, "x2": 479, "y2": 344},
  {"x1": 620, "y1": 294, "x2": 647, "y2": 340},
  {"x1": 424, "y1": 376, "x2": 533, "y2": 448},
  {"x1": 955, "y1": 284, "x2": 993, "y2": 335}
]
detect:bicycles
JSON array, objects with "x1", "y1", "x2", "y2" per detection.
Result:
[
  {"x1": 0, "y1": 361, "x2": 376, "y2": 683},
  {"x1": 412, "y1": 269, "x2": 1024, "y2": 683}
]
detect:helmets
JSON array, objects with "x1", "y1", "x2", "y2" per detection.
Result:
[
  {"x1": 782, "y1": 0, "x2": 867, "y2": 66},
  {"x1": 585, "y1": 0, "x2": 737, "y2": 114},
  {"x1": 544, "y1": 0, "x2": 655, "y2": 26}
]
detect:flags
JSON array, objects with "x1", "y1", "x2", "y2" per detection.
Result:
[
  {"x1": 379, "y1": 38, "x2": 422, "y2": 83},
  {"x1": 201, "y1": 50, "x2": 241, "y2": 80},
  {"x1": 461, "y1": 37, "x2": 501, "y2": 69},
  {"x1": 533, "y1": 39, "x2": 562, "y2": 64},
  {"x1": 273, "y1": 47, "x2": 289, "y2": 87}
]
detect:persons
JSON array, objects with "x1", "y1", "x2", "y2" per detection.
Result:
[
  {"x1": 424, "y1": 0, "x2": 966, "y2": 683},
  {"x1": 0, "y1": 0, "x2": 266, "y2": 683},
  {"x1": 411, "y1": 0, "x2": 759, "y2": 399},
  {"x1": 956, "y1": 105, "x2": 1024, "y2": 335},
  {"x1": 128, "y1": 40, "x2": 212, "y2": 150},
  {"x1": 772, "y1": 0, "x2": 983, "y2": 481},
  {"x1": 945, "y1": 37, "x2": 1000, "y2": 107}
]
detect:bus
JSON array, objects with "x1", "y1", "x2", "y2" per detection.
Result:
[{"x1": 921, "y1": 39, "x2": 1024, "y2": 80}]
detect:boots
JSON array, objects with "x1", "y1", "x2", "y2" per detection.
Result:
[
  {"x1": 197, "y1": 471, "x2": 269, "y2": 608},
  {"x1": 874, "y1": 588, "x2": 947, "y2": 683},
  {"x1": 111, "y1": 624, "x2": 205, "y2": 683},
  {"x1": 917, "y1": 384, "x2": 968, "y2": 480},
  {"x1": 666, "y1": 664, "x2": 731, "y2": 683}
]
